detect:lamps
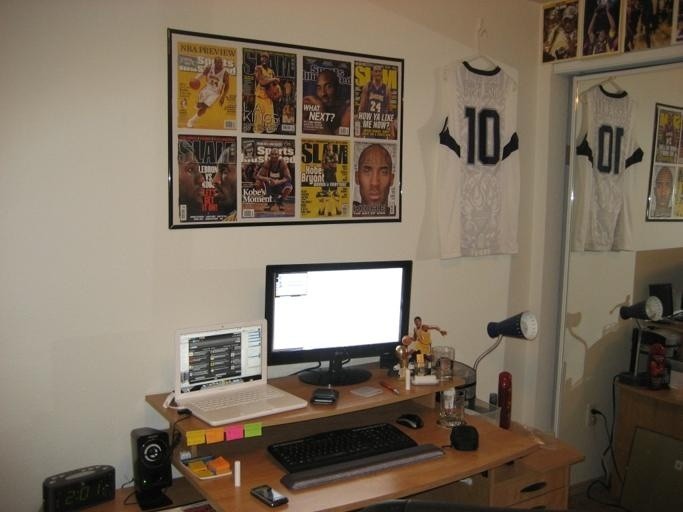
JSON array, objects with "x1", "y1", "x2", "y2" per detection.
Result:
[
  {"x1": 618, "y1": 295, "x2": 666, "y2": 389},
  {"x1": 465, "y1": 308, "x2": 539, "y2": 410}
]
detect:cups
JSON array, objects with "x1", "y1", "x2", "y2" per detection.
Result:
[
  {"x1": 439, "y1": 390, "x2": 466, "y2": 427},
  {"x1": 430, "y1": 345, "x2": 454, "y2": 384}
]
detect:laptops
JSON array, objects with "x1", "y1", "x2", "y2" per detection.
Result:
[{"x1": 173, "y1": 319, "x2": 309, "y2": 427}]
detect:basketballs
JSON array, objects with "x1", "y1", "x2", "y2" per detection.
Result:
[
  {"x1": 402, "y1": 336, "x2": 411, "y2": 345},
  {"x1": 269, "y1": 84, "x2": 281, "y2": 97},
  {"x1": 189, "y1": 78, "x2": 201, "y2": 90}
]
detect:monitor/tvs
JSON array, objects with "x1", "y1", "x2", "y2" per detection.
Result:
[{"x1": 264, "y1": 259, "x2": 413, "y2": 387}]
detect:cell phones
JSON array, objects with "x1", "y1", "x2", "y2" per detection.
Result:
[{"x1": 250, "y1": 483, "x2": 289, "y2": 509}]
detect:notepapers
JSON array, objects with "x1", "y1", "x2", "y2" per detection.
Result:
[{"x1": 188, "y1": 456, "x2": 230, "y2": 477}]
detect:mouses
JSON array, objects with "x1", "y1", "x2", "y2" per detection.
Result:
[{"x1": 397, "y1": 413, "x2": 424, "y2": 429}]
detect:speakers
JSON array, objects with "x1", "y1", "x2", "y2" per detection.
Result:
[{"x1": 130, "y1": 427, "x2": 173, "y2": 492}]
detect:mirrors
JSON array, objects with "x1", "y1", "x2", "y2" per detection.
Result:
[{"x1": 550, "y1": 61, "x2": 683, "y2": 511}]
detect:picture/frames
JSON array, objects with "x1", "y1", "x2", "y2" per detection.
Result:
[
  {"x1": 164, "y1": 25, "x2": 404, "y2": 230},
  {"x1": 645, "y1": 102, "x2": 682, "y2": 221},
  {"x1": 537, "y1": 0, "x2": 682, "y2": 65}
]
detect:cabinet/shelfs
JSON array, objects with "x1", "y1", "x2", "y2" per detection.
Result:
[
  {"x1": 70, "y1": 357, "x2": 585, "y2": 511},
  {"x1": 611, "y1": 318, "x2": 683, "y2": 512}
]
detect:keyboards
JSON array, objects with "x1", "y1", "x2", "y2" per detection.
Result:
[{"x1": 268, "y1": 422, "x2": 420, "y2": 473}]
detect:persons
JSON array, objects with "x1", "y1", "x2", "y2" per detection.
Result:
[
  {"x1": 405, "y1": 318, "x2": 448, "y2": 363},
  {"x1": 650, "y1": 166, "x2": 674, "y2": 218},
  {"x1": 543, "y1": 0, "x2": 660, "y2": 60},
  {"x1": 179, "y1": 50, "x2": 395, "y2": 222},
  {"x1": 663, "y1": 113, "x2": 674, "y2": 158}
]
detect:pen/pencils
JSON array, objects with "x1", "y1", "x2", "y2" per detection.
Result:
[{"x1": 379, "y1": 380, "x2": 400, "y2": 394}]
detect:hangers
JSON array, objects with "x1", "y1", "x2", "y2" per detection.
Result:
[
  {"x1": 599, "y1": 76, "x2": 624, "y2": 93},
  {"x1": 465, "y1": 28, "x2": 498, "y2": 69}
]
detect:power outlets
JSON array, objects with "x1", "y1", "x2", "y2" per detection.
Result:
[{"x1": 584, "y1": 400, "x2": 596, "y2": 429}]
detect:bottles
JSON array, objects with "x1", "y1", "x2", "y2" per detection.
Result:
[
  {"x1": 497, "y1": 371, "x2": 512, "y2": 428},
  {"x1": 646, "y1": 343, "x2": 665, "y2": 390}
]
